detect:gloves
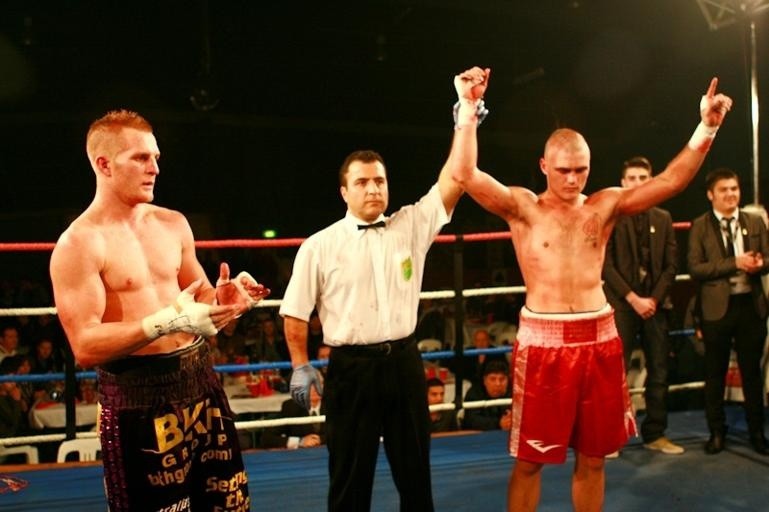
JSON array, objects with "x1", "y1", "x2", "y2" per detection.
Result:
[
  {"x1": 213, "y1": 271, "x2": 263, "y2": 320},
  {"x1": 142, "y1": 291, "x2": 218, "y2": 341},
  {"x1": 290, "y1": 365, "x2": 323, "y2": 410},
  {"x1": 453, "y1": 97, "x2": 489, "y2": 129}
]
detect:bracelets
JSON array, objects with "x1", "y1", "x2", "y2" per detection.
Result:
[
  {"x1": 457, "y1": 99, "x2": 477, "y2": 128},
  {"x1": 688, "y1": 121, "x2": 718, "y2": 154}
]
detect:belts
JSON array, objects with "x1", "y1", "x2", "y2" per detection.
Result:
[{"x1": 352, "y1": 336, "x2": 415, "y2": 355}]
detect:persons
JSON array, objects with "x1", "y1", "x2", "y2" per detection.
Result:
[
  {"x1": 603, "y1": 156, "x2": 685, "y2": 456},
  {"x1": 451, "y1": 67, "x2": 733, "y2": 512},
  {"x1": 49, "y1": 108, "x2": 273, "y2": 512},
  {"x1": 415, "y1": 284, "x2": 528, "y2": 437},
  {"x1": 198, "y1": 285, "x2": 331, "y2": 453},
  {"x1": 611, "y1": 288, "x2": 767, "y2": 421},
  {"x1": 687, "y1": 170, "x2": 767, "y2": 455},
  {"x1": 1, "y1": 286, "x2": 103, "y2": 458},
  {"x1": 279, "y1": 96, "x2": 492, "y2": 510}
]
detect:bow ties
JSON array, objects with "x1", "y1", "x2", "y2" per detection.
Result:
[{"x1": 357, "y1": 221, "x2": 386, "y2": 230}]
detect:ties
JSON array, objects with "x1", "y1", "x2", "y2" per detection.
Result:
[{"x1": 722, "y1": 217, "x2": 738, "y2": 286}]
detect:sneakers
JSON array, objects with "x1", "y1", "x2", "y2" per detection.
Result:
[{"x1": 643, "y1": 438, "x2": 684, "y2": 455}]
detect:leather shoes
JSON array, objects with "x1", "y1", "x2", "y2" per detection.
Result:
[
  {"x1": 750, "y1": 433, "x2": 769, "y2": 456},
  {"x1": 707, "y1": 425, "x2": 728, "y2": 454}
]
